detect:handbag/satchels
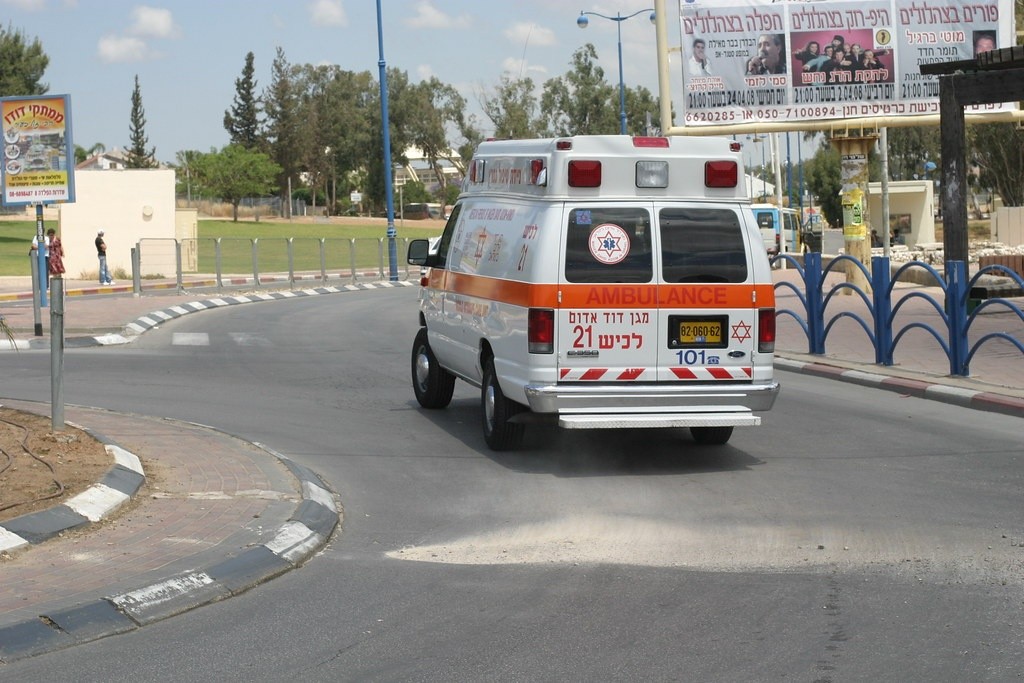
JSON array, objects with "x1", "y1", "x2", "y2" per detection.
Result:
[{"x1": 29, "y1": 249, "x2": 33, "y2": 256}]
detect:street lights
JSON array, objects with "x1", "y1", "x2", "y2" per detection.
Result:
[
  {"x1": 577, "y1": 8, "x2": 656, "y2": 134},
  {"x1": 746, "y1": 134, "x2": 768, "y2": 203}
]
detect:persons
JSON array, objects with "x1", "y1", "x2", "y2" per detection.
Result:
[
  {"x1": 95, "y1": 230, "x2": 116, "y2": 284},
  {"x1": 975, "y1": 33, "x2": 995, "y2": 56},
  {"x1": 870, "y1": 229, "x2": 882, "y2": 247},
  {"x1": 688, "y1": 40, "x2": 711, "y2": 75},
  {"x1": 48, "y1": 229, "x2": 65, "y2": 278},
  {"x1": 31, "y1": 227, "x2": 50, "y2": 291},
  {"x1": 747, "y1": 33, "x2": 786, "y2": 74},
  {"x1": 797, "y1": 33, "x2": 885, "y2": 82},
  {"x1": 890, "y1": 229, "x2": 904, "y2": 247}
]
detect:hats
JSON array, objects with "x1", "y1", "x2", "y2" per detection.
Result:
[{"x1": 98, "y1": 231, "x2": 104, "y2": 235}]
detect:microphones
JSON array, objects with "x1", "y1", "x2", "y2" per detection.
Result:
[{"x1": 749, "y1": 52, "x2": 766, "y2": 73}]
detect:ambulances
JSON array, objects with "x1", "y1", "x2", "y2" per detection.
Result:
[{"x1": 407, "y1": 134, "x2": 781, "y2": 452}]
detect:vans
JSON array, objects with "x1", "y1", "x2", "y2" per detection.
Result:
[{"x1": 750, "y1": 204, "x2": 824, "y2": 259}]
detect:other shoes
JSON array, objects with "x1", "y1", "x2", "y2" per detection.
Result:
[
  {"x1": 102, "y1": 281, "x2": 110, "y2": 285},
  {"x1": 109, "y1": 280, "x2": 116, "y2": 285}
]
